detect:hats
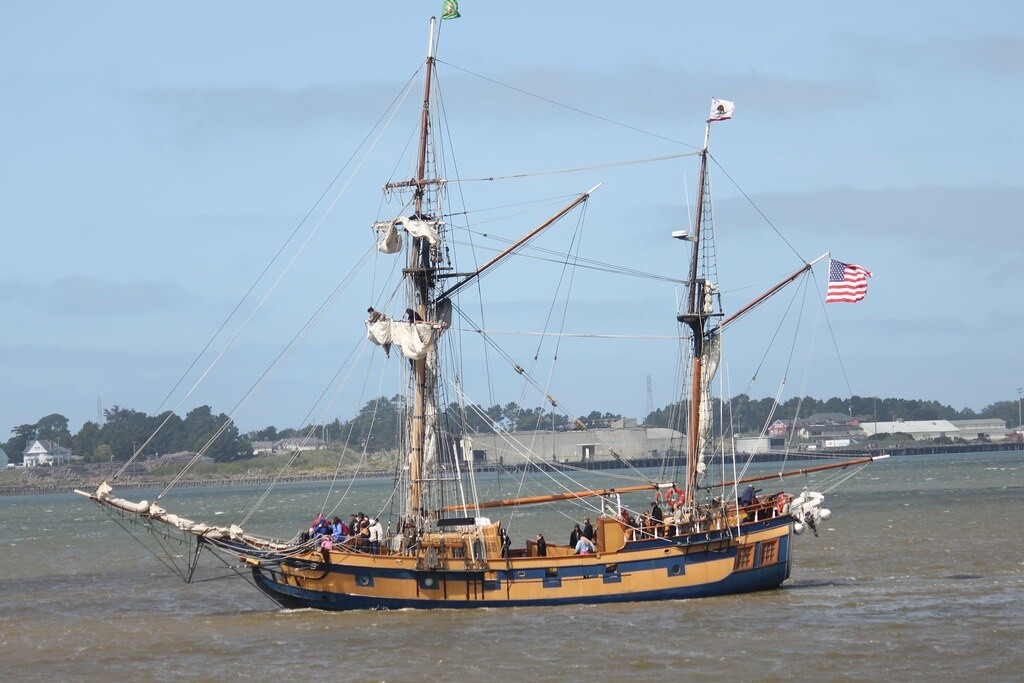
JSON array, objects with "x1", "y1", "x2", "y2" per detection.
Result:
[{"x1": 651, "y1": 502, "x2": 656, "y2": 507}]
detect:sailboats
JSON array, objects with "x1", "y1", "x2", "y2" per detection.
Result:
[
  {"x1": 74, "y1": 14, "x2": 803, "y2": 607},
  {"x1": 590, "y1": 120, "x2": 890, "y2": 551}
]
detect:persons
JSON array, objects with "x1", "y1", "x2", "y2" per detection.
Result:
[
  {"x1": 668, "y1": 504, "x2": 683, "y2": 537},
  {"x1": 710, "y1": 496, "x2": 721, "y2": 508},
  {"x1": 626, "y1": 518, "x2": 639, "y2": 535},
  {"x1": 640, "y1": 502, "x2": 662, "y2": 537},
  {"x1": 367, "y1": 307, "x2": 384, "y2": 323},
  {"x1": 501, "y1": 529, "x2": 511, "y2": 558},
  {"x1": 536, "y1": 534, "x2": 546, "y2": 557},
  {"x1": 574, "y1": 533, "x2": 597, "y2": 554},
  {"x1": 569, "y1": 524, "x2": 584, "y2": 548},
  {"x1": 311, "y1": 512, "x2": 382, "y2": 555},
  {"x1": 741, "y1": 484, "x2": 755, "y2": 522},
  {"x1": 583, "y1": 516, "x2": 593, "y2": 541},
  {"x1": 406, "y1": 308, "x2": 422, "y2": 326}
]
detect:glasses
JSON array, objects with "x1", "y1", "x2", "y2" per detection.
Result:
[{"x1": 537, "y1": 537, "x2": 538, "y2": 538}]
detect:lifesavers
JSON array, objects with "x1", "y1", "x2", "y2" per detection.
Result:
[
  {"x1": 666, "y1": 487, "x2": 684, "y2": 507},
  {"x1": 778, "y1": 493, "x2": 784, "y2": 511}
]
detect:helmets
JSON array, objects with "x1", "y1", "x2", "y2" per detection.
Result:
[
  {"x1": 749, "y1": 484, "x2": 754, "y2": 488},
  {"x1": 582, "y1": 516, "x2": 590, "y2": 521}
]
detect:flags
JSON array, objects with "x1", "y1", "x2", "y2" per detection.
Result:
[
  {"x1": 441, "y1": 0, "x2": 461, "y2": 19},
  {"x1": 710, "y1": 99, "x2": 734, "y2": 121},
  {"x1": 826, "y1": 259, "x2": 872, "y2": 304}
]
